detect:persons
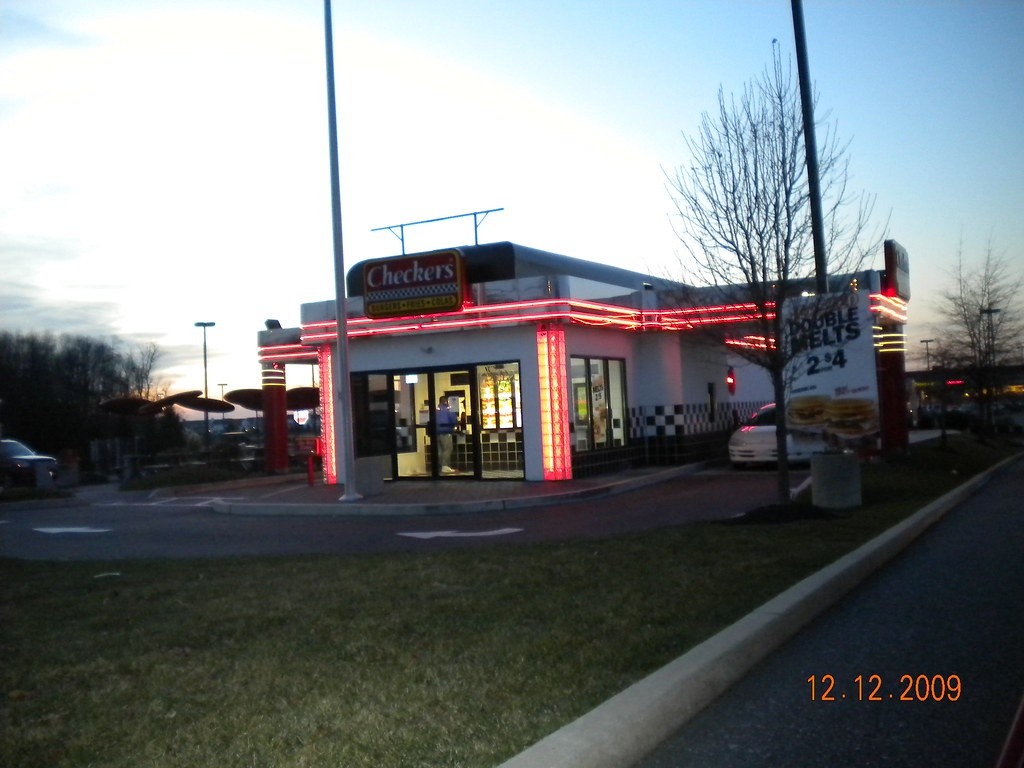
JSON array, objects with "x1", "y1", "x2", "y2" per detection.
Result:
[{"x1": 436, "y1": 396, "x2": 459, "y2": 473}]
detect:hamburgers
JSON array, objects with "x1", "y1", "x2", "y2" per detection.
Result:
[
  {"x1": 788, "y1": 396, "x2": 828, "y2": 423},
  {"x1": 825, "y1": 399, "x2": 875, "y2": 433}
]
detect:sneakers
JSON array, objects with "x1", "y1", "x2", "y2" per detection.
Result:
[{"x1": 442, "y1": 466, "x2": 455, "y2": 474}]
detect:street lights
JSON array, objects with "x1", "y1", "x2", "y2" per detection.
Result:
[
  {"x1": 920, "y1": 339, "x2": 935, "y2": 403},
  {"x1": 196, "y1": 320, "x2": 215, "y2": 464}
]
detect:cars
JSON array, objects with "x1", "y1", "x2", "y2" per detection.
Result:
[
  {"x1": 727, "y1": 401, "x2": 846, "y2": 471},
  {"x1": 1, "y1": 438, "x2": 57, "y2": 488}
]
